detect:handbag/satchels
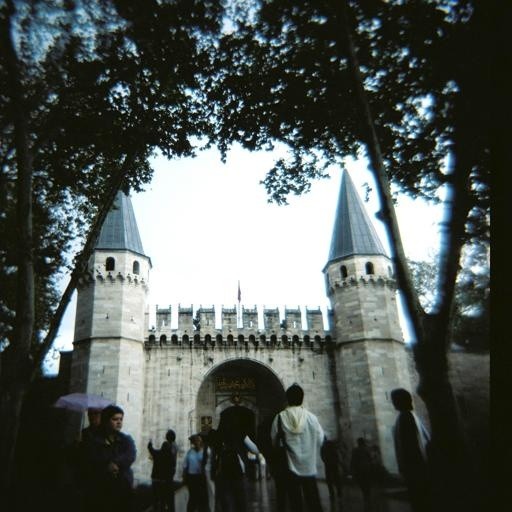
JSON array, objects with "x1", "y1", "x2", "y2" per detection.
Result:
[{"x1": 275, "y1": 431, "x2": 289, "y2": 478}]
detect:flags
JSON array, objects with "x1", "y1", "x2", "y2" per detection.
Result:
[{"x1": 237, "y1": 281, "x2": 242, "y2": 303}]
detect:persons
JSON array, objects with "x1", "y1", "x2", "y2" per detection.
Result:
[
  {"x1": 52, "y1": 406, "x2": 177, "y2": 511},
  {"x1": 320, "y1": 438, "x2": 381, "y2": 503},
  {"x1": 390, "y1": 387, "x2": 442, "y2": 512},
  {"x1": 181, "y1": 382, "x2": 325, "y2": 512}
]
